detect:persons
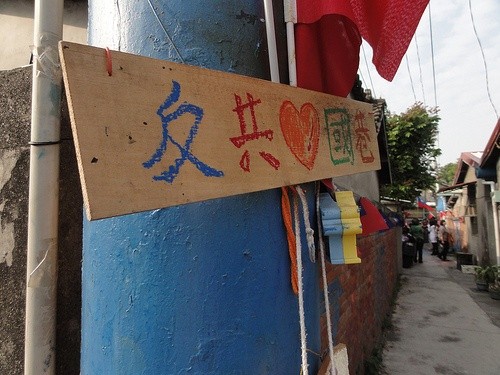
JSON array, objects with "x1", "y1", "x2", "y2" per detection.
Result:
[
  {"x1": 427, "y1": 220, "x2": 440, "y2": 255},
  {"x1": 437, "y1": 220, "x2": 451, "y2": 261},
  {"x1": 422, "y1": 220, "x2": 429, "y2": 242},
  {"x1": 409, "y1": 218, "x2": 423, "y2": 263}
]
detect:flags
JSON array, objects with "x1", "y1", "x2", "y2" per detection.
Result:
[
  {"x1": 283, "y1": 0, "x2": 429, "y2": 190},
  {"x1": 417, "y1": 197, "x2": 435, "y2": 211}
]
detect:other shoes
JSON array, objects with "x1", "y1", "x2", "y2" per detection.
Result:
[
  {"x1": 438, "y1": 256, "x2": 442, "y2": 259},
  {"x1": 419, "y1": 261, "x2": 423, "y2": 263}
]
